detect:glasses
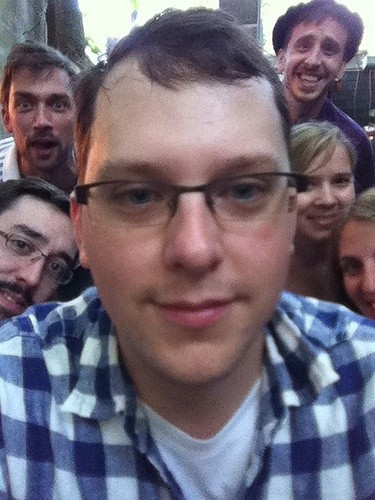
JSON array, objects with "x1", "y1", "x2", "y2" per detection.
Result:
[
  {"x1": 75, "y1": 170, "x2": 311, "y2": 228},
  {"x1": 0, "y1": 230, "x2": 75, "y2": 285}
]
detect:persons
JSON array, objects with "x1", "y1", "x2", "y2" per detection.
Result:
[
  {"x1": 283, "y1": 121, "x2": 354, "y2": 305},
  {"x1": 272, "y1": 0, "x2": 375, "y2": 197},
  {"x1": 338, "y1": 188, "x2": 375, "y2": 322},
  {"x1": 1, "y1": 178, "x2": 81, "y2": 326},
  {"x1": 0, "y1": 42, "x2": 82, "y2": 196},
  {"x1": 0, "y1": 9, "x2": 375, "y2": 500}
]
